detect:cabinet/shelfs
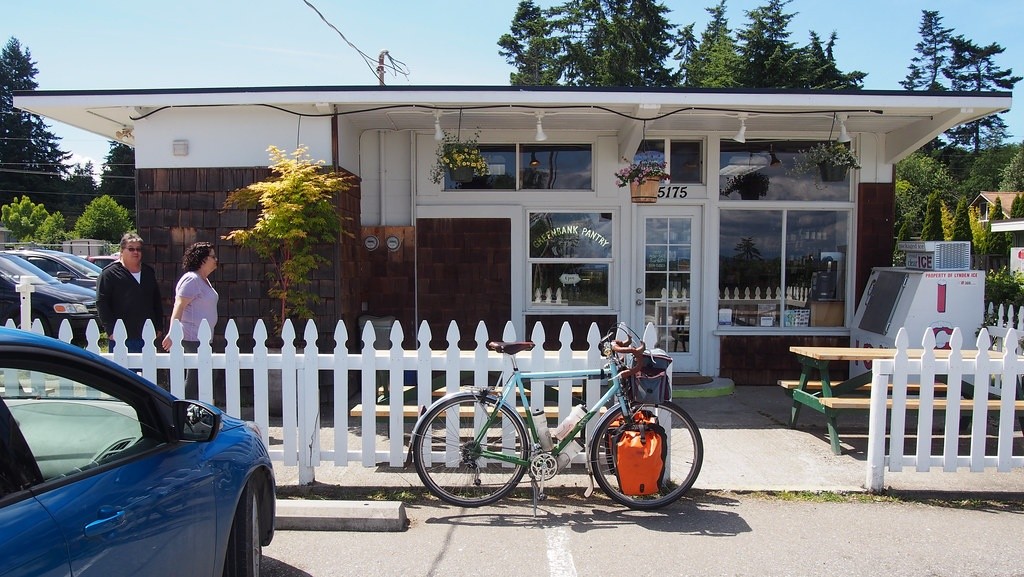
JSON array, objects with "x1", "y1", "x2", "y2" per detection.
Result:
[{"x1": 762, "y1": 225, "x2": 832, "y2": 288}]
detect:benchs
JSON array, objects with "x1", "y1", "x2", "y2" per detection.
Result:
[
  {"x1": 819, "y1": 397, "x2": 1024, "y2": 410},
  {"x1": 777, "y1": 380, "x2": 949, "y2": 391},
  {"x1": 379, "y1": 385, "x2": 583, "y2": 396},
  {"x1": 349, "y1": 404, "x2": 612, "y2": 417}
]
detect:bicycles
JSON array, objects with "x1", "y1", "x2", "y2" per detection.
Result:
[{"x1": 408, "y1": 323, "x2": 704, "y2": 511}]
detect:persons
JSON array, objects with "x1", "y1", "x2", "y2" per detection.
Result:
[
  {"x1": 162, "y1": 241, "x2": 219, "y2": 399},
  {"x1": 95, "y1": 232, "x2": 164, "y2": 374}
]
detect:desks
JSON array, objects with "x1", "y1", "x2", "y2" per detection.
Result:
[
  {"x1": 788, "y1": 347, "x2": 1024, "y2": 454},
  {"x1": 673, "y1": 310, "x2": 785, "y2": 355}
]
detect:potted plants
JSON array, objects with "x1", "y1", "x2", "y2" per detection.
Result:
[{"x1": 218, "y1": 143, "x2": 360, "y2": 415}]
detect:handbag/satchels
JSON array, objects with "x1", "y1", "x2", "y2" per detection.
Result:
[
  {"x1": 605, "y1": 410, "x2": 668, "y2": 495},
  {"x1": 626, "y1": 348, "x2": 673, "y2": 405}
]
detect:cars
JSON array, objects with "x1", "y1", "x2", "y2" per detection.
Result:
[
  {"x1": 0, "y1": 248, "x2": 121, "y2": 338},
  {"x1": 0, "y1": 324, "x2": 277, "y2": 577}
]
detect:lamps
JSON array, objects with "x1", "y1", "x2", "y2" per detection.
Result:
[
  {"x1": 432, "y1": 109, "x2": 445, "y2": 140},
  {"x1": 836, "y1": 113, "x2": 852, "y2": 143},
  {"x1": 733, "y1": 111, "x2": 747, "y2": 143},
  {"x1": 534, "y1": 110, "x2": 547, "y2": 142}
]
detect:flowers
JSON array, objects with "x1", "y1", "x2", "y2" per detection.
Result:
[
  {"x1": 779, "y1": 142, "x2": 862, "y2": 176},
  {"x1": 614, "y1": 154, "x2": 672, "y2": 188},
  {"x1": 424, "y1": 123, "x2": 490, "y2": 184}
]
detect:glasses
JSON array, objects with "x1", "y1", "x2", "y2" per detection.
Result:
[
  {"x1": 207, "y1": 255, "x2": 217, "y2": 260},
  {"x1": 122, "y1": 247, "x2": 142, "y2": 252}
]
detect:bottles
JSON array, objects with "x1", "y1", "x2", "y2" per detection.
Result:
[
  {"x1": 531, "y1": 408, "x2": 554, "y2": 452},
  {"x1": 554, "y1": 404, "x2": 589, "y2": 441},
  {"x1": 551, "y1": 438, "x2": 585, "y2": 475}
]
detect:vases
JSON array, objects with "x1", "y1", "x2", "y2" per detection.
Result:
[
  {"x1": 630, "y1": 175, "x2": 660, "y2": 203},
  {"x1": 446, "y1": 164, "x2": 474, "y2": 181},
  {"x1": 819, "y1": 161, "x2": 848, "y2": 182}
]
